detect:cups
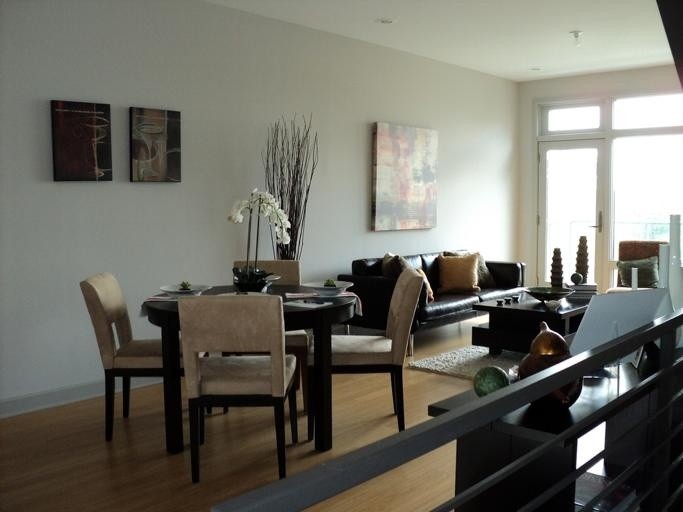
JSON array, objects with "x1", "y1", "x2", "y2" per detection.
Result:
[{"x1": 497, "y1": 296, "x2": 519, "y2": 304}]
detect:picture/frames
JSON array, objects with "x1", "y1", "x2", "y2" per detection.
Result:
[{"x1": 628, "y1": 342, "x2": 660, "y2": 369}]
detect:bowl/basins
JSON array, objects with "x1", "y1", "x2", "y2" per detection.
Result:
[
  {"x1": 544, "y1": 300, "x2": 562, "y2": 311},
  {"x1": 160, "y1": 284, "x2": 213, "y2": 297},
  {"x1": 523, "y1": 287, "x2": 575, "y2": 301},
  {"x1": 302, "y1": 282, "x2": 354, "y2": 295},
  {"x1": 218, "y1": 292, "x2": 270, "y2": 296}
]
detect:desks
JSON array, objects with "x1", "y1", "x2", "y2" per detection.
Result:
[{"x1": 144, "y1": 282, "x2": 359, "y2": 455}]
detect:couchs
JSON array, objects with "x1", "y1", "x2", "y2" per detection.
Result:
[{"x1": 336, "y1": 249, "x2": 529, "y2": 357}]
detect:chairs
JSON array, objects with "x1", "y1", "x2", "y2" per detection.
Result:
[
  {"x1": 604, "y1": 239, "x2": 668, "y2": 296},
  {"x1": 218, "y1": 259, "x2": 308, "y2": 415},
  {"x1": 79, "y1": 269, "x2": 208, "y2": 450},
  {"x1": 171, "y1": 291, "x2": 298, "y2": 485},
  {"x1": 304, "y1": 264, "x2": 425, "y2": 444}
]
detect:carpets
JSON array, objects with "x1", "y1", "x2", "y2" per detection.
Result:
[{"x1": 406, "y1": 345, "x2": 527, "y2": 382}]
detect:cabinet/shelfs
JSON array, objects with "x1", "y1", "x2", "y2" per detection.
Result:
[{"x1": 426, "y1": 357, "x2": 683, "y2": 512}]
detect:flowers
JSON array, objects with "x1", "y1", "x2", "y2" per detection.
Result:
[{"x1": 225, "y1": 188, "x2": 293, "y2": 275}]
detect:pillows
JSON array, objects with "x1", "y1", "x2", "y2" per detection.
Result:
[
  {"x1": 382, "y1": 252, "x2": 435, "y2": 305},
  {"x1": 614, "y1": 255, "x2": 662, "y2": 290},
  {"x1": 436, "y1": 251, "x2": 482, "y2": 295},
  {"x1": 442, "y1": 252, "x2": 494, "y2": 288}
]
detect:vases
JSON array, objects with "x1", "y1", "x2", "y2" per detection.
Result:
[{"x1": 232, "y1": 270, "x2": 269, "y2": 294}]
